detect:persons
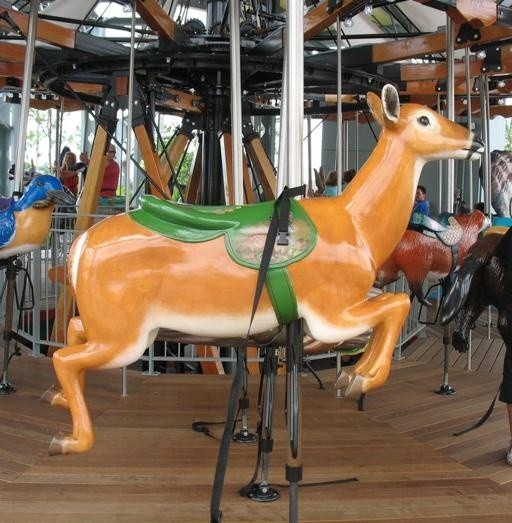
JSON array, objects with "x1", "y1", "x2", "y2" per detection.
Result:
[
  {"x1": 340, "y1": 168, "x2": 355, "y2": 190},
  {"x1": 178, "y1": 184, "x2": 187, "y2": 202},
  {"x1": 53, "y1": 146, "x2": 70, "y2": 168},
  {"x1": 497, "y1": 353, "x2": 511, "y2": 467},
  {"x1": 80, "y1": 142, "x2": 120, "y2": 200},
  {"x1": 52, "y1": 152, "x2": 79, "y2": 198},
  {"x1": 323, "y1": 169, "x2": 338, "y2": 196}
]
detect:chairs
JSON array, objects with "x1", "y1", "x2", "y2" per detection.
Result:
[{"x1": 507, "y1": 446, "x2": 512, "y2": 465}]
